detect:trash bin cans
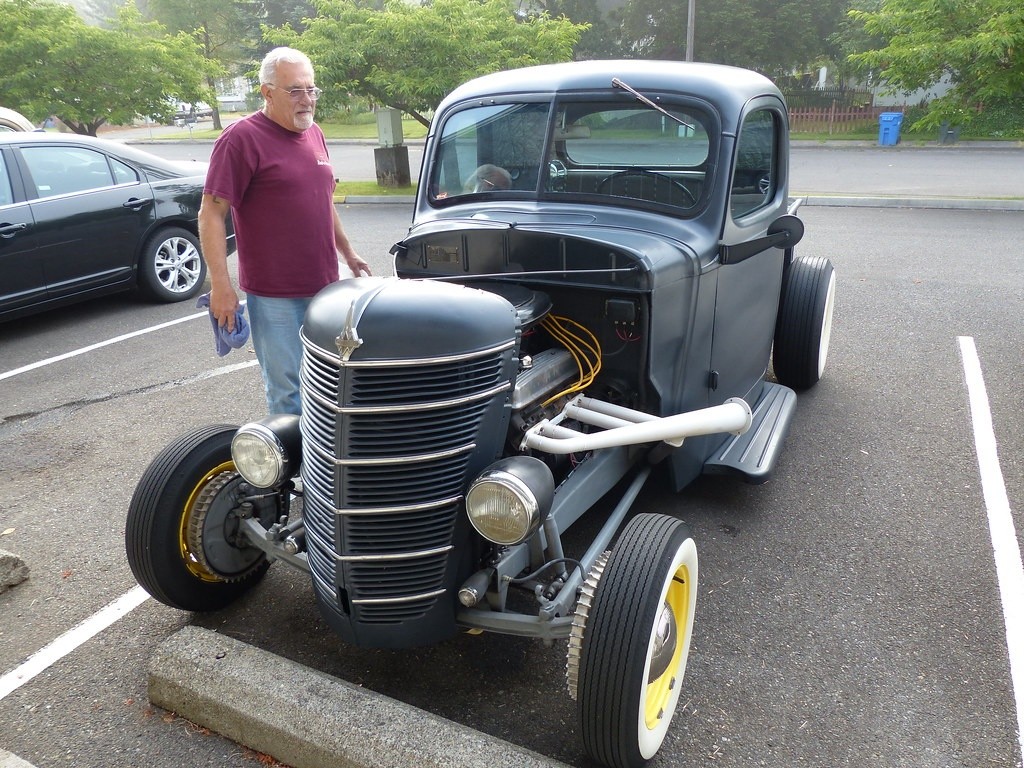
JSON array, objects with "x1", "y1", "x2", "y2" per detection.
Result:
[
  {"x1": 878, "y1": 111, "x2": 904, "y2": 145},
  {"x1": 939, "y1": 113, "x2": 962, "y2": 146}
]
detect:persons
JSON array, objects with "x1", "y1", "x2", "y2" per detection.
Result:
[
  {"x1": 198, "y1": 47, "x2": 372, "y2": 416},
  {"x1": 462, "y1": 163, "x2": 513, "y2": 194}
]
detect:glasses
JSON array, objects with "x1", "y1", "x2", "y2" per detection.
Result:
[{"x1": 265, "y1": 82, "x2": 322, "y2": 101}]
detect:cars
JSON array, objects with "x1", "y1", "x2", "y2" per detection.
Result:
[
  {"x1": 126, "y1": 59, "x2": 835, "y2": 768},
  {"x1": 0, "y1": 131, "x2": 236, "y2": 322},
  {"x1": 0, "y1": 108, "x2": 38, "y2": 136}
]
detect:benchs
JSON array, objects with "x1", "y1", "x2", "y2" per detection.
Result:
[{"x1": 542, "y1": 169, "x2": 708, "y2": 196}]
